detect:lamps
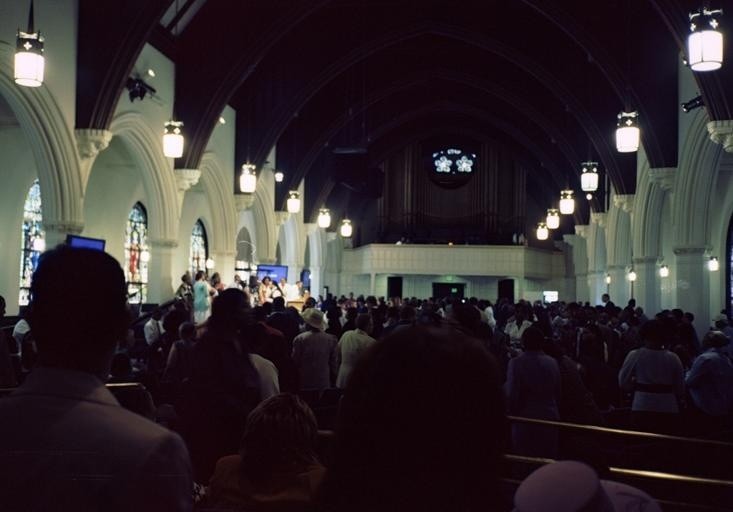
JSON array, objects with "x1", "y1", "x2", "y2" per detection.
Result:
[
  {"x1": 10, "y1": 1, "x2": 48, "y2": 89},
  {"x1": 238, "y1": 0, "x2": 357, "y2": 238},
  {"x1": 158, "y1": 1, "x2": 188, "y2": 158}
]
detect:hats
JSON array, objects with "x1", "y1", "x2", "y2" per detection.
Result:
[
  {"x1": 711, "y1": 313, "x2": 728, "y2": 322},
  {"x1": 301, "y1": 307, "x2": 329, "y2": 331},
  {"x1": 505, "y1": 458, "x2": 664, "y2": 512}
]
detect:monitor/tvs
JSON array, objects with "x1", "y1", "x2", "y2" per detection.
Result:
[
  {"x1": 67, "y1": 234, "x2": 106, "y2": 251},
  {"x1": 300, "y1": 269, "x2": 310, "y2": 287},
  {"x1": 257, "y1": 264, "x2": 288, "y2": 283}
]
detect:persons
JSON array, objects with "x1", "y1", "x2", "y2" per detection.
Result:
[{"x1": 0, "y1": 246, "x2": 732, "y2": 510}]
